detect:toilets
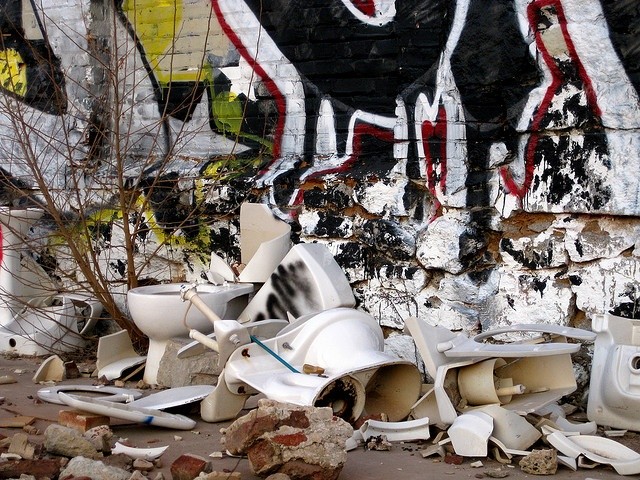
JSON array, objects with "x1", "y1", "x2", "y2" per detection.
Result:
[{"x1": 127, "y1": 282, "x2": 255, "y2": 383}]
